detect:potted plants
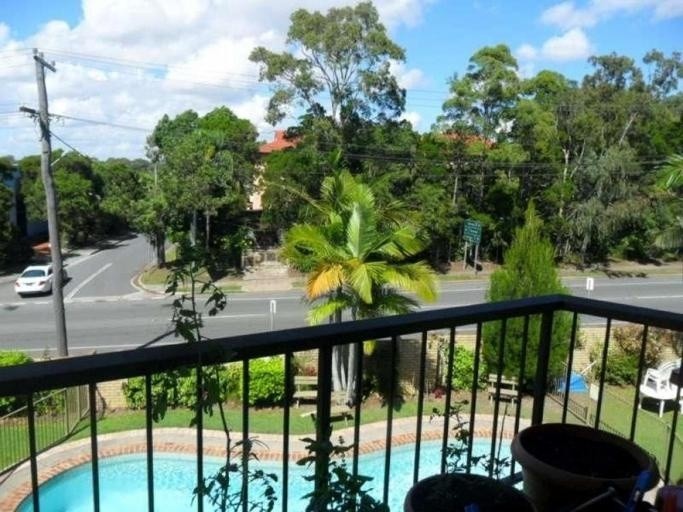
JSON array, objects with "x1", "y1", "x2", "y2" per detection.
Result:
[{"x1": 404, "y1": 400, "x2": 538, "y2": 512}]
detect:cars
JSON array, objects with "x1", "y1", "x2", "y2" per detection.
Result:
[{"x1": 12, "y1": 262, "x2": 67, "y2": 296}]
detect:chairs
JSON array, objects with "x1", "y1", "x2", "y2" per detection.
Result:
[{"x1": 643, "y1": 357, "x2": 681, "y2": 393}]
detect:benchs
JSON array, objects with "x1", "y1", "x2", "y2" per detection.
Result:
[
  {"x1": 488, "y1": 373, "x2": 518, "y2": 408},
  {"x1": 330, "y1": 390, "x2": 350, "y2": 425},
  {"x1": 293, "y1": 376, "x2": 318, "y2": 408}
]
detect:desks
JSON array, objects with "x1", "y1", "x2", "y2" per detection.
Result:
[{"x1": 637, "y1": 382, "x2": 679, "y2": 418}]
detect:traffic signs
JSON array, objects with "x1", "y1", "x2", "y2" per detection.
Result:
[{"x1": 463, "y1": 220, "x2": 481, "y2": 244}]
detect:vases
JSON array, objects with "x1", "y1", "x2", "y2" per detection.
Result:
[{"x1": 511, "y1": 423, "x2": 658, "y2": 512}]
